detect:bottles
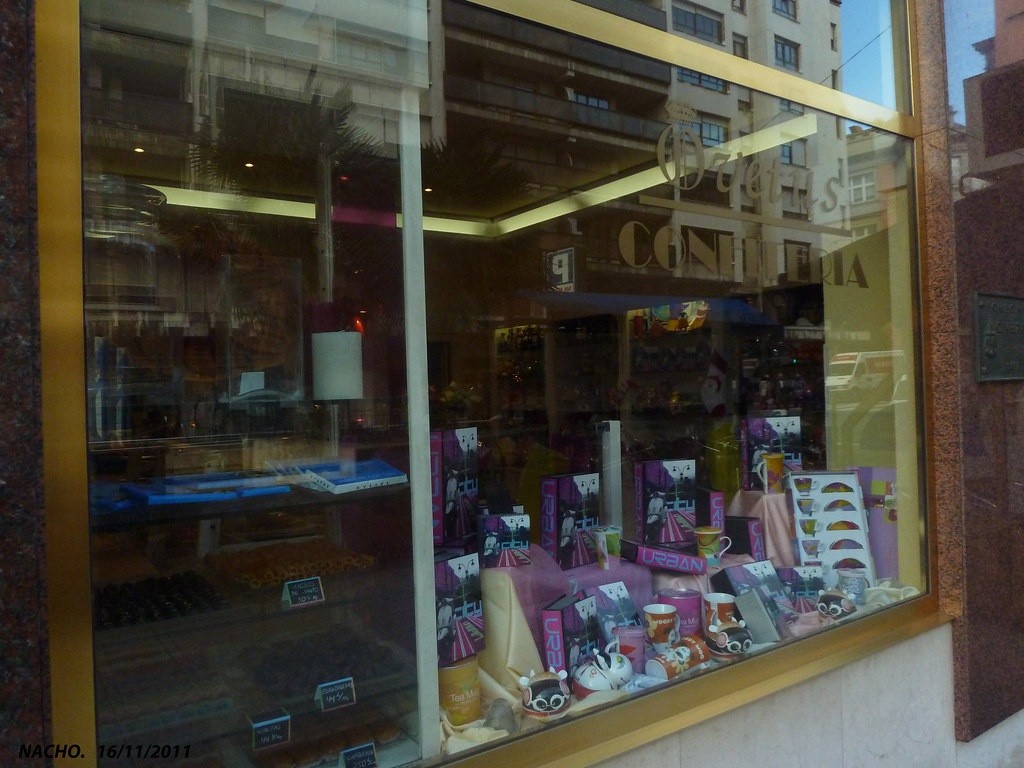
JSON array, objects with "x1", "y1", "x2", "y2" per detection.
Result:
[
  {"x1": 758, "y1": 372, "x2": 808, "y2": 417},
  {"x1": 498, "y1": 324, "x2": 541, "y2": 354}
]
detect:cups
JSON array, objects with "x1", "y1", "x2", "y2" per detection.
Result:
[
  {"x1": 647, "y1": 636, "x2": 711, "y2": 680},
  {"x1": 589, "y1": 524, "x2": 623, "y2": 570},
  {"x1": 755, "y1": 453, "x2": 784, "y2": 495},
  {"x1": 659, "y1": 587, "x2": 700, "y2": 638},
  {"x1": 643, "y1": 605, "x2": 681, "y2": 642},
  {"x1": 570, "y1": 640, "x2": 632, "y2": 692},
  {"x1": 690, "y1": 525, "x2": 732, "y2": 567},
  {"x1": 701, "y1": 590, "x2": 735, "y2": 637},
  {"x1": 612, "y1": 626, "x2": 645, "y2": 673}
]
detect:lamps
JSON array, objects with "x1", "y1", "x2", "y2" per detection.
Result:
[{"x1": 309, "y1": 332, "x2": 366, "y2": 459}]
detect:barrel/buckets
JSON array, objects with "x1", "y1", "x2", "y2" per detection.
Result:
[{"x1": 438, "y1": 654, "x2": 480, "y2": 727}]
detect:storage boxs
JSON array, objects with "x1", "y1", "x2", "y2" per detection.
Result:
[{"x1": 785, "y1": 471, "x2": 876, "y2": 593}]
